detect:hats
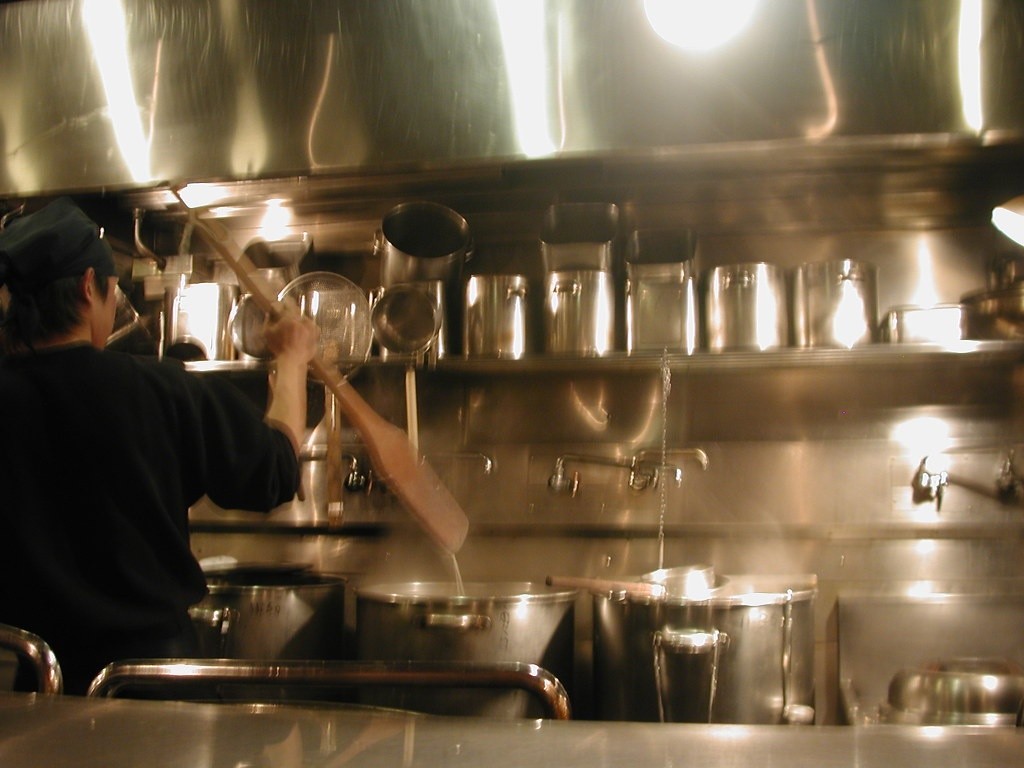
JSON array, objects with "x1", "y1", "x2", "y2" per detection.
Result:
[{"x1": 2, "y1": 196, "x2": 118, "y2": 277}]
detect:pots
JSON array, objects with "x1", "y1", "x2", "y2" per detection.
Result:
[
  {"x1": 876, "y1": 654, "x2": 1024, "y2": 729},
  {"x1": 588, "y1": 576, "x2": 821, "y2": 727},
  {"x1": 190, "y1": 562, "x2": 345, "y2": 702},
  {"x1": 355, "y1": 580, "x2": 575, "y2": 724}
]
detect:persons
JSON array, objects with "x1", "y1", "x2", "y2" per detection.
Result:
[{"x1": 1, "y1": 196, "x2": 325, "y2": 701}]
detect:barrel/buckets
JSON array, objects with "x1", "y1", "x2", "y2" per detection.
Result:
[{"x1": 100, "y1": 200, "x2": 879, "y2": 360}]
